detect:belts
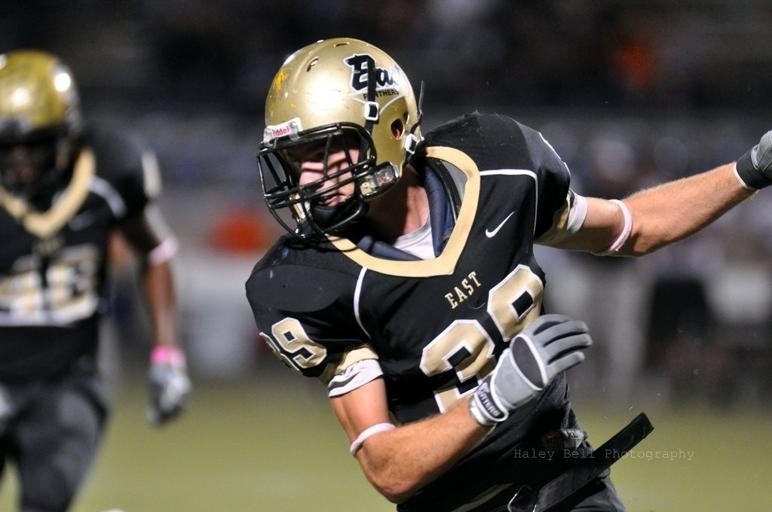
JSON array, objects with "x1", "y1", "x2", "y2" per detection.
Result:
[{"x1": 476, "y1": 411, "x2": 655, "y2": 512}]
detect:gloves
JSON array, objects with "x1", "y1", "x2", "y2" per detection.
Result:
[
  {"x1": 142, "y1": 344, "x2": 196, "y2": 427},
  {"x1": 732, "y1": 122, "x2": 772, "y2": 196},
  {"x1": 467, "y1": 307, "x2": 595, "y2": 427}
]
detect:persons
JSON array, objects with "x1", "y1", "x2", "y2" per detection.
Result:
[
  {"x1": 1, "y1": 50, "x2": 193, "y2": 511},
  {"x1": 243, "y1": 37, "x2": 771, "y2": 511}
]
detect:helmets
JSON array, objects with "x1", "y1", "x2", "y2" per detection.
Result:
[
  {"x1": 256, "y1": 32, "x2": 428, "y2": 228},
  {"x1": 1, "y1": 47, "x2": 84, "y2": 146}
]
